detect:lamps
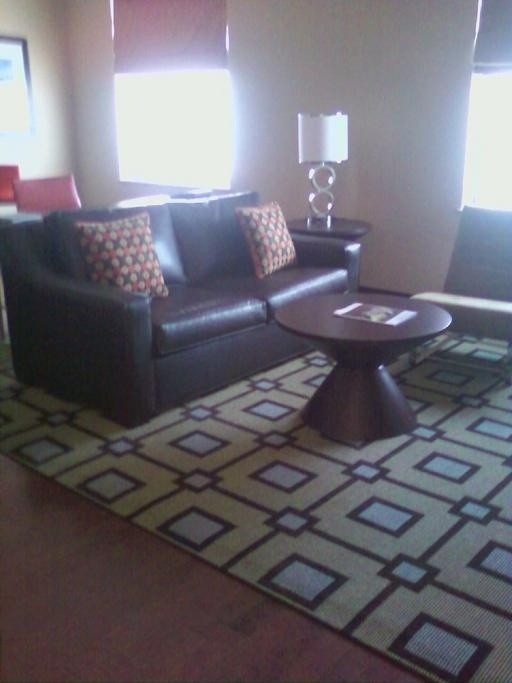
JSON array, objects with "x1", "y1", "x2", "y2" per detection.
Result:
[{"x1": 291, "y1": 102, "x2": 357, "y2": 235}]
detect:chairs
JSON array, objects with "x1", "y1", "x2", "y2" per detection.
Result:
[{"x1": 0, "y1": 160, "x2": 89, "y2": 230}]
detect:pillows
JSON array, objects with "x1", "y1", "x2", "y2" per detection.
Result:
[
  {"x1": 236, "y1": 199, "x2": 305, "y2": 287},
  {"x1": 78, "y1": 206, "x2": 175, "y2": 308}
]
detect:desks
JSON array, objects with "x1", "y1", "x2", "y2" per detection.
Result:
[
  {"x1": 272, "y1": 285, "x2": 462, "y2": 448},
  {"x1": 279, "y1": 209, "x2": 374, "y2": 285}
]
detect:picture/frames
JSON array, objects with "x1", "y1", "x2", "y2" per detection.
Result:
[{"x1": 0, "y1": 29, "x2": 53, "y2": 138}]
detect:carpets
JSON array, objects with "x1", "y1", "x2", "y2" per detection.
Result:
[{"x1": 1, "y1": 317, "x2": 512, "y2": 683}]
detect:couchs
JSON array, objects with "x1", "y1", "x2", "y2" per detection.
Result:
[
  {"x1": 3, "y1": 193, "x2": 373, "y2": 418},
  {"x1": 409, "y1": 199, "x2": 512, "y2": 356}
]
